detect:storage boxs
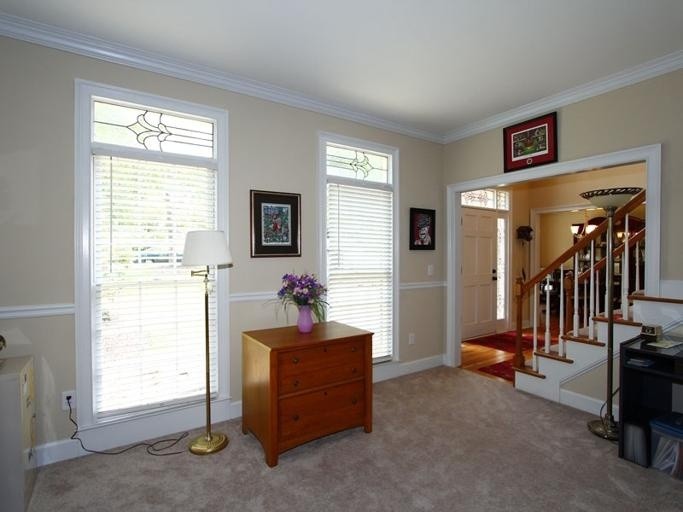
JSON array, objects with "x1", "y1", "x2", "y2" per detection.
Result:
[{"x1": 648, "y1": 418, "x2": 683, "y2": 478}]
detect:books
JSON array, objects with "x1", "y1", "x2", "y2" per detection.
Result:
[
  {"x1": 627, "y1": 358, "x2": 656, "y2": 368},
  {"x1": 649, "y1": 436, "x2": 683, "y2": 479}
]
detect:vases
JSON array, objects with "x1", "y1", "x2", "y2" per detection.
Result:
[{"x1": 295, "y1": 304, "x2": 314, "y2": 334}]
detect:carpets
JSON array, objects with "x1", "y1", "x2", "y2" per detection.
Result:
[
  {"x1": 477, "y1": 357, "x2": 521, "y2": 382},
  {"x1": 461, "y1": 330, "x2": 559, "y2": 354}
]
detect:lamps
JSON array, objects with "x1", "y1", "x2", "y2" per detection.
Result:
[
  {"x1": 577, "y1": 186, "x2": 642, "y2": 442},
  {"x1": 179, "y1": 229, "x2": 234, "y2": 456}
]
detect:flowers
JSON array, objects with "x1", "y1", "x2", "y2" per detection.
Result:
[{"x1": 275, "y1": 270, "x2": 331, "y2": 326}]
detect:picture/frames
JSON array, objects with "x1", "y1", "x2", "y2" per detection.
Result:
[
  {"x1": 249, "y1": 189, "x2": 301, "y2": 258},
  {"x1": 503, "y1": 111, "x2": 558, "y2": 173},
  {"x1": 409, "y1": 207, "x2": 436, "y2": 250}
]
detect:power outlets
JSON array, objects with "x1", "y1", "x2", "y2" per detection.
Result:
[{"x1": 60, "y1": 389, "x2": 76, "y2": 412}]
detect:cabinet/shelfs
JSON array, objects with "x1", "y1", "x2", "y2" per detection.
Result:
[
  {"x1": 240, "y1": 320, "x2": 376, "y2": 467},
  {"x1": 618, "y1": 330, "x2": 683, "y2": 469},
  {"x1": 0, "y1": 354, "x2": 38, "y2": 512}
]
